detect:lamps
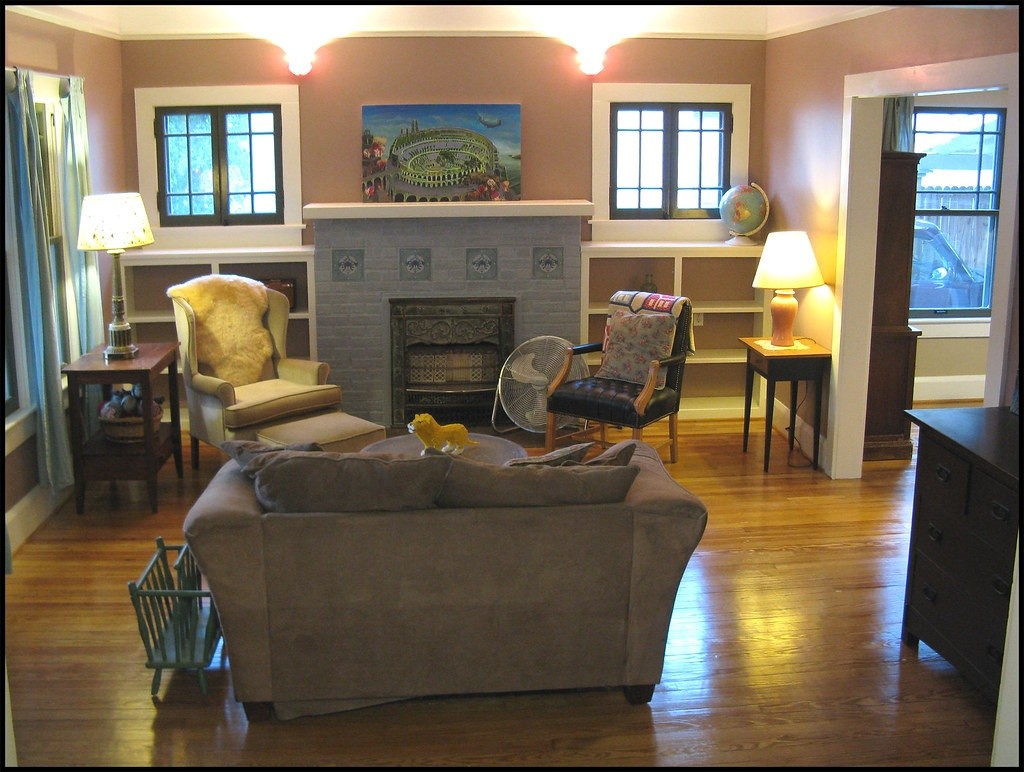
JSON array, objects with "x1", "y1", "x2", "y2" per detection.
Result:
[
  {"x1": 752, "y1": 230, "x2": 826, "y2": 346},
  {"x1": 76, "y1": 191, "x2": 155, "y2": 362}
]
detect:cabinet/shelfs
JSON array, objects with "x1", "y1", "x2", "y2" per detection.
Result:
[
  {"x1": 579, "y1": 241, "x2": 775, "y2": 423},
  {"x1": 120, "y1": 242, "x2": 319, "y2": 433},
  {"x1": 900, "y1": 405, "x2": 1019, "y2": 708},
  {"x1": 864, "y1": 150, "x2": 928, "y2": 460}
]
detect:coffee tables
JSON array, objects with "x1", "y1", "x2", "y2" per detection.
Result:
[{"x1": 360, "y1": 431, "x2": 528, "y2": 465}]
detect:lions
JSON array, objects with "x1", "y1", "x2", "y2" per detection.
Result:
[{"x1": 407, "y1": 413, "x2": 480, "y2": 456}]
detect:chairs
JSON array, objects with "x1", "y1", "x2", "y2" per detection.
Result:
[
  {"x1": 544, "y1": 290, "x2": 696, "y2": 464},
  {"x1": 166, "y1": 274, "x2": 387, "y2": 471}
]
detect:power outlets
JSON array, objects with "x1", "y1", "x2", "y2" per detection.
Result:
[{"x1": 693, "y1": 314, "x2": 704, "y2": 327}]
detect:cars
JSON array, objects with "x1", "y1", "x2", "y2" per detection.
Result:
[{"x1": 909, "y1": 219, "x2": 984, "y2": 308}]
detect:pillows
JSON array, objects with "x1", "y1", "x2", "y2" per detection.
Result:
[
  {"x1": 594, "y1": 308, "x2": 676, "y2": 391},
  {"x1": 219, "y1": 439, "x2": 326, "y2": 469},
  {"x1": 560, "y1": 441, "x2": 638, "y2": 467},
  {"x1": 420, "y1": 446, "x2": 641, "y2": 510},
  {"x1": 240, "y1": 449, "x2": 453, "y2": 513},
  {"x1": 503, "y1": 441, "x2": 596, "y2": 468}
]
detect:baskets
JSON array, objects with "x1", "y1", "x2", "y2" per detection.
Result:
[{"x1": 99, "y1": 399, "x2": 162, "y2": 442}]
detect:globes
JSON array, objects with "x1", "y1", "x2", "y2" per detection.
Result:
[{"x1": 718, "y1": 182, "x2": 770, "y2": 246}]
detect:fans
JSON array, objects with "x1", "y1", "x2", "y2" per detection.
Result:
[{"x1": 492, "y1": 334, "x2": 591, "y2": 438}]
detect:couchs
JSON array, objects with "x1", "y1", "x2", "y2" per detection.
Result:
[{"x1": 182, "y1": 437, "x2": 709, "y2": 723}]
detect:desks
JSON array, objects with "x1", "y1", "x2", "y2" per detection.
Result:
[
  {"x1": 61, "y1": 341, "x2": 184, "y2": 515},
  {"x1": 738, "y1": 336, "x2": 831, "y2": 474}
]
